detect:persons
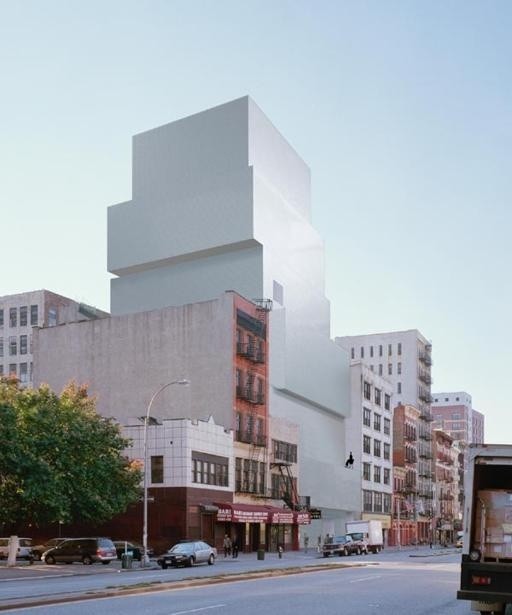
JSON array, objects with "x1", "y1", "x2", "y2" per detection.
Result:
[
  {"x1": 222, "y1": 531, "x2": 232, "y2": 560},
  {"x1": 327, "y1": 534, "x2": 334, "y2": 543},
  {"x1": 303, "y1": 531, "x2": 310, "y2": 555},
  {"x1": 231, "y1": 532, "x2": 239, "y2": 558},
  {"x1": 316, "y1": 533, "x2": 322, "y2": 554},
  {"x1": 324, "y1": 533, "x2": 329, "y2": 544}
]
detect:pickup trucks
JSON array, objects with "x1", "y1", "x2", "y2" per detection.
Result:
[{"x1": 323, "y1": 535, "x2": 363, "y2": 556}]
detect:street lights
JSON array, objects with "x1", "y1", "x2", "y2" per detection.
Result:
[{"x1": 141, "y1": 379, "x2": 190, "y2": 567}]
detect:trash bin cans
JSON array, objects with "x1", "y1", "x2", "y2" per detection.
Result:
[
  {"x1": 122, "y1": 555, "x2": 132, "y2": 569},
  {"x1": 258, "y1": 549, "x2": 264, "y2": 560}
]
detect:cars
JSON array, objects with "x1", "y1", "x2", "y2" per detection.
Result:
[
  {"x1": 157, "y1": 541, "x2": 217, "y2": 569},
  {"x1": 1, "y1": 536, "x2": 155, "y2": 565},
  {"x1": 455, "y1": 537, "x2": 463, "y2": 548}
]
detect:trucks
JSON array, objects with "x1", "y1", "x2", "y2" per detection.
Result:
[
  {"x1": 455, "y1": 443, "x2": 511, "y2": 614},
  {"x1": 344, "y1": 520, "x2": 383, "y2": 553}
]
